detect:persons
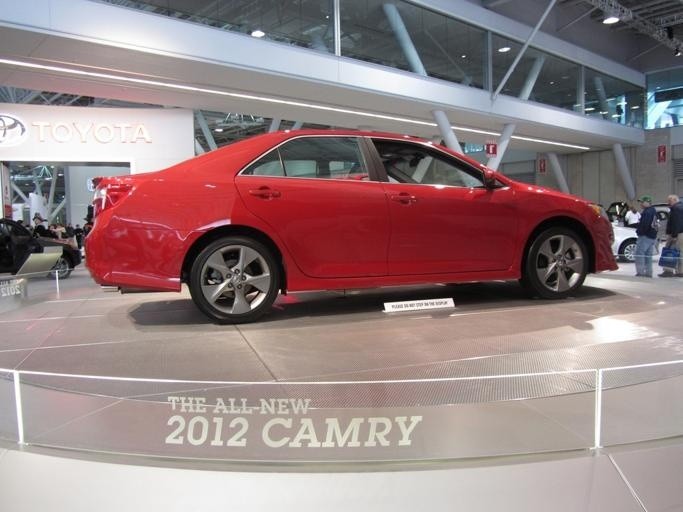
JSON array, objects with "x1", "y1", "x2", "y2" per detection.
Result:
[
  {"x1": 11, "y1": 215, "x2": 89, "y2": 252},
  {"x1": 657, "y1": 194, "x2": 682, "y2": 277},
  {"x1": 624, "y1": 204, "x2": 641, "y2": 225},
  {"x1": 633, "y1": 196, "x2": 658, "y2": 278}
]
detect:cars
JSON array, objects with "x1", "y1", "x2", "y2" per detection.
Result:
[
  {"x1": 639, "y1": 203, "x2": 670, "y2": 239},
  {"x1": 82, "y1": 128, "x2": 619, "y2": 324},
  {"x1": 0, "y1": 218, "x2": 81, "y2": 279},
  {"x1": 611, "y1": 221, "x2": 638, "y2": 263},
  {"x1": 606, "y1": 202, "x2": 629, "y2": 225}
]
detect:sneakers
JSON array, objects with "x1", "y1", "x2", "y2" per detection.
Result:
[{"x1": 634, "y1": 271, "x2": 682, "y2": 277}]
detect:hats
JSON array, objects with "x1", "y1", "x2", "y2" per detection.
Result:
[{"x1": 637, "y1": 195, "x2": 651, "y2": 202}]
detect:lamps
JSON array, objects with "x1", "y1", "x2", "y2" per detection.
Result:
[
  {"x1": 601, "y1": 7, "x2": 620, "y2": 27},
  {"x1": 249, "y1": 18, "x2": 266, "y2": 38},
  {"x1": 495, "y1": 37, "x2": 511, "y2": 53}
]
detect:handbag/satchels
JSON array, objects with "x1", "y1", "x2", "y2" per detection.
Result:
[{"x1": 658, "y1": 243, "x2": 679, "y2": 268}]
